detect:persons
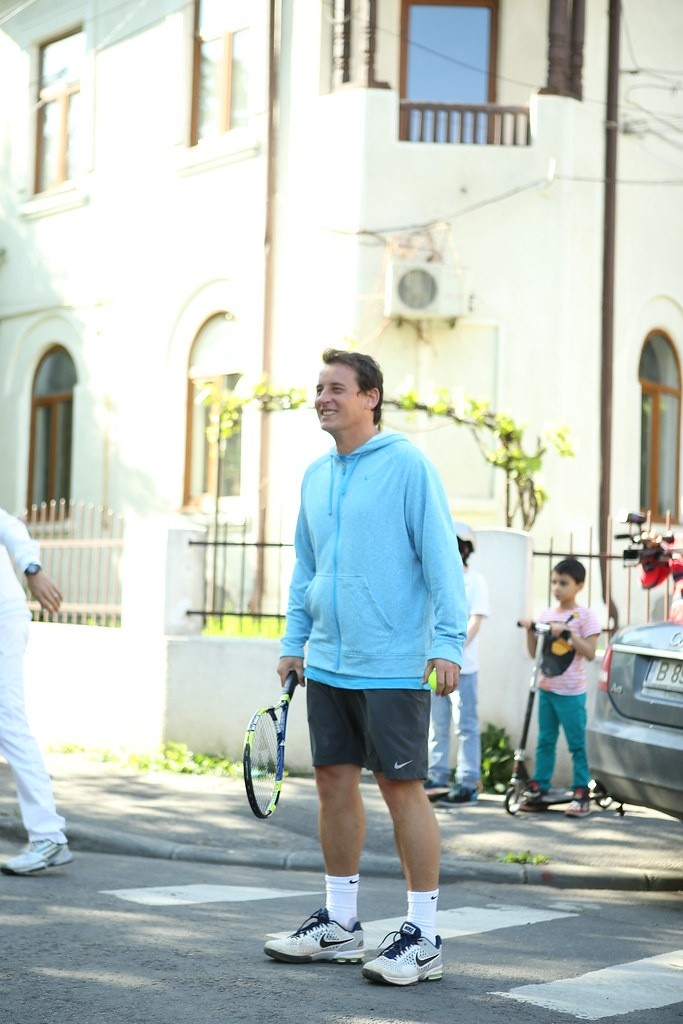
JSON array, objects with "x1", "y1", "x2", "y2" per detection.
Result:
[
  {"x1": 519, "y1": 558, "x2": 601, "y2": 817},
  {"x1": 265, "y1": 349, "x2": 468, "y2": 985},
  {"x1": 0, "y1": 508, "x2": 74, "y2": 873},
  {"x1": 423, "y1": 535, "x2": 489, "y2": 806}
]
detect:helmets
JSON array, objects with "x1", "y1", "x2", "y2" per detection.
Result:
[{"x1": 452, "y1": 520, "x2": 477, "y2": 552}]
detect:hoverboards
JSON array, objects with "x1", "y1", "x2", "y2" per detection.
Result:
[{"x1": 505, "y1": 622, "x2": 614, "y2": 816}]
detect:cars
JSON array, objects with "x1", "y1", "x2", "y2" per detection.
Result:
[{"x1": 583, "y1": 621, "x2": 682, "y2": 825}]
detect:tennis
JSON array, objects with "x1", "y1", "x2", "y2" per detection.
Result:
[{"x1": 427, "y1": 670, "x2": 437, "y2": 692}]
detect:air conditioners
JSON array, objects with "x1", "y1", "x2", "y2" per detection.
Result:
[{"x1": 383, "y1": 258, "x2": 470, "y2": 321}]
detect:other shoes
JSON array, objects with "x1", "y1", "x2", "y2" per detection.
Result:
[
  {"x1": 520, "y1": 782, "x2": 550, "y2": 812},
  {"x1": 567, "y1": 788, "x2": 591, "y2": 816}
]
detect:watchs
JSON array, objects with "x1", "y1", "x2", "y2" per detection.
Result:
[{"x1": 24, "y1": 564, "x2": 42, "y2": 576}]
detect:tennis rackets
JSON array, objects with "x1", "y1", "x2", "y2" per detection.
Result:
[{"x1": 242, "y1": 666, "x2": 306, "y2": 820}]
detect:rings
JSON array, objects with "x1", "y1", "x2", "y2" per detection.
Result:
[{"x1": 52, "y1": 592, "x2": 57, "y2": 598}]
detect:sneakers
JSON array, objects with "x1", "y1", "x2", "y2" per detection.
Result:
[
  {"x1": 264, "y1": 908, "x2": 364, "y2": 964},
  {"x1": 436, "y1": 783, "x2": 478, "y2": 805},
  {"x1": 423, "y1": 779, "x2": 446, "y2": 790},
  {"x1": 361, "y1": 920, "x2": 444, "y2": 984},
  {"x1": 0, "y1": 841, "x2": 73, "y2": 875}
]
